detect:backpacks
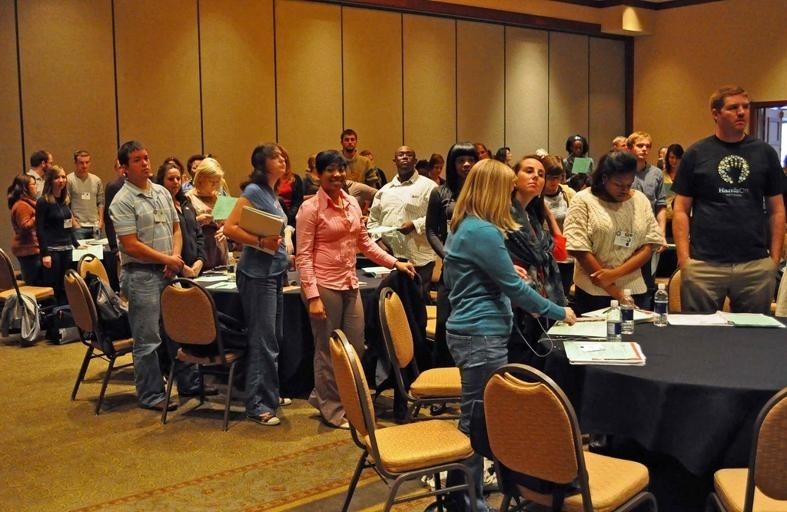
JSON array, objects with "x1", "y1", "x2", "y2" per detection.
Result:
[
  {"x1": 181, "y1": 311, "x2": 248, "y2": 354},
  {"x1": 82, "y1": 271, "x2": 131, "y2": 340}
]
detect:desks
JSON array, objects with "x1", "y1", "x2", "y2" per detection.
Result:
[
  {"x1": 535, "y1": 312, "x2": 787, "y2": 509},
  {"x1": 184, "y1": 262, "x2": 436, "y2": 399}
]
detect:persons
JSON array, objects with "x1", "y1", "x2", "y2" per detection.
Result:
[
  {"x1": 225, "y1": 129, "x2": 512, "y2": 429},
  {"x1": 505, "y1": 156, "x2": 567, "y2": 369},
  {"x1": 563, "y1": 151, "x2": 665, "y2": 318},
  {"x1": 102, "y1": 140, "x2": 231, "y2": 411},
  {"x1": 443, "y1": 159, "x2": 576, "y2": 512},
  {"x1": 6, "y1": 148, "x2": 105, "y2": 345},
  {"x1": 539, "y1": 130, "x2": 685, "y2": 288},
  {"x1": 670, "y1": 85, "x2": 787, "y2": 314}
]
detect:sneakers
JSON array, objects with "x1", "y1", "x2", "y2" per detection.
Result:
[
  {"x1": 278, "y1": 397, "x2": 292, "y2": 406},
  {"x1": 198, "y1": 384, "x2": 218, "y2": 396},
  {"x1": 246, "y1": 411, "x2": 280, "y2": 425},
  {"x1": 154, "y1": 398, "x2": 178, "y2": 412}
]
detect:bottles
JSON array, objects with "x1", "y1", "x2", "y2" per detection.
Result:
[
  {"x1": 93, "y1": 222, "x2": 100, "y2": 241},
  {"x1": 620, "y1": 288, "x2": 634, "y2": 337},
  {"x1": 605, "y1": 298, "x2": 621, "y2": 339},
  {"x1": 650, "y1": 281, "x2": 670, "y2": 327},
  {"x1": 225, "y1": 251, "x2": 236, "y2": 283}
]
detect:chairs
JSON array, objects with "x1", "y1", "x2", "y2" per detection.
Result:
[
  {"x1": 378, "y1": 287, "x2": 461, "y2": 422},
  {"x1": 62, "y1": 268, "x2": 134, "y2": 416},
  {"x1": 158, "y1": 277, "x2": 245, "y2": 432},
  {"x1": 0, "y1": 247, "x2": 54, "y2": 341},
  {"x1": 327, "y1": 328, "x2": 479, "y2": 511},
  {"x1": 704, "y1": 385, "x2": 787, "y2": 511},
  {"x1": 77, "y1": 253, "x2": 111, "y2": 290},
  {"x1": 484, "y1": 363, "x2": 658, "y2": 511}
]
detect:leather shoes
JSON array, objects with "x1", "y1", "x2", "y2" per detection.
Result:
[{"x1": 431, "y1": 404, "x2": 444, "y2": 415}]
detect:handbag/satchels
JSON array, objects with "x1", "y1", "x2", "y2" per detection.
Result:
[
  {"x1": 44, "y1": 304, "x2": 82, "y2": 341},
  {"x1": 424, "y1": 456, "x2": 500, "y2": 495}
]
